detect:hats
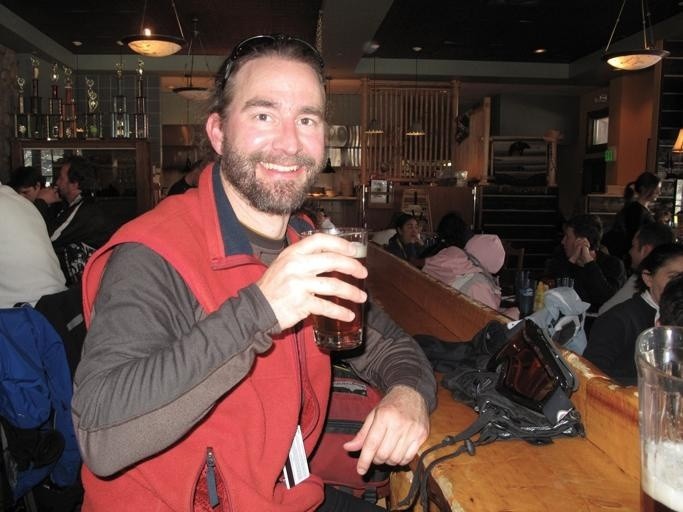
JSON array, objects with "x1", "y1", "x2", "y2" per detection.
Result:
[
  {"x1": 544, "y1": 286, "x2": 591, "y2": 320},
  {"x1": 464, "y1": 233, "x2": 505, "y2": 274}
]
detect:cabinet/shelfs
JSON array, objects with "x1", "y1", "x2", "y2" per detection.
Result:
[
  {"x1": 366, "y1": 242, "x2": 683, "y2": 512},
  {"x1": 306, "y1": 194, "x2": 360, "y2": 229},
  {"x1": 9, "y1": 138, "x2": 154, "y2": 225}
]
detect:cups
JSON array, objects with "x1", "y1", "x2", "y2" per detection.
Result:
[
  {"x1": 522, "y1": 280, "x2": 536, "y2": 313},
  {"x1": 634, "y1": 325, "x2": 683, "y2": 511},
  {"x1": 557, "y1": 277, "x2": 575, "y2": 287},
  {"x1": 299, "y1": 226, "x2": 369, "y2": 351},
  {"x1": 519, "y1": 269, "x2": 530, "y2": 286}
]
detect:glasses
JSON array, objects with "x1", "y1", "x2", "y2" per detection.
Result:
[{"x1": 222, "y1": 33, "x2": 320, "y2": 88}]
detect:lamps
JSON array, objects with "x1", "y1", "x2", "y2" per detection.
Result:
[
  {"x1": 600, "y1": 0, "x2": 672, "y2": 72},
  {"x1": 672, "y1": 128, "x2": 683, "y2": 153},
  {"x1": 362, "y1": 44, "x2": 385, "y2": 134},
  {"x1": 405, "y1": 47, "x2": 426, "y2": 136},
  {"x1": 172, "y1": 18, "x2": 214, "y2": 102},
  {"x1": 119, "y1": 0, "x2": 188, "y2": 59}
]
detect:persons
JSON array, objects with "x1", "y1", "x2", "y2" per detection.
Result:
[
  {"x1": 421, "y1": 234, "x2": 520, "y2": 320},
  {"x1": 12, "y1": 166, "x2": 42, "y2": 203},
  {"x1": 419, "y1": 212, "x2": 469, "y2": 260},
  {"x1": 69, "y1": 34, "x2": 437, "y2": 512},
  {"x1": 32, "y1": 157, "x2": 93, "y2": 244},
  {"x1": 624, "y1": 171, "x2": 661, "y2": 247},
  {"x1": 545, "y1": 214, "x2": 619, "y2": 291},
  {"x1": 598, "y1": 222, "x2": 674, "y2": 317},
  {"x1": 657, "y1": 270, "x2": 683, "y2": 328},
  {"x1": 167, "y1": 153, "x2": 213, "y2": 195},
  {"x1": 388, "y1": 215, "x2": 425, "y2": 261},
  {"x1": 588, "y1": 242, "x2": 683, "y2": 386},
  {"x1": 0, "y1": 183, "x2": 69, "y2": 335}
]
